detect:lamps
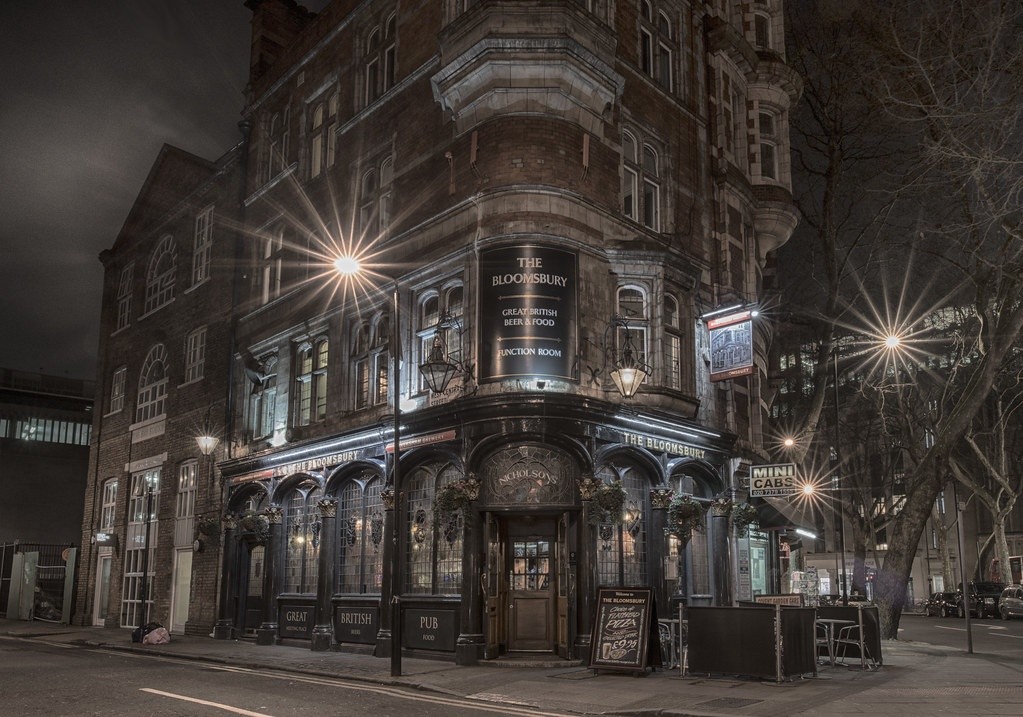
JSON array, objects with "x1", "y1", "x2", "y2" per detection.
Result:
[
  {"x1": 420, "y1": 312, "x2": 471, "y2": 394},
  {"x1": 195, "y1": 401, "x2": 239, "y2": 455},
  {"x1": 587, "y1": 314, "x2": 655, "y2": 402}
]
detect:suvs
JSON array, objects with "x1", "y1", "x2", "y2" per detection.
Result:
[
  {"x1": 998, "y1": 584, "x2": 1023, "y2": 620},
  {"x1": 954, "y1": 581, "x2": 1004, "y2": 619}
]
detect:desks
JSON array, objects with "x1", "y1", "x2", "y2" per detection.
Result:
[
  {"x1": 818, "y1": 618, "x2": 856, "y2": 667},
  {"x1": 659, "y1": 619, "x2": 688, "y2": 670}
]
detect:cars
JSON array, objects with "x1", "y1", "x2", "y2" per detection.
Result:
[{"x1": 925, "y1": 592, "x2": 958, "y2": 617}]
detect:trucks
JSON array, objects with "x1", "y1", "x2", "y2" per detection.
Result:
[{"x1": 990, "y1": 555, "x2": 1023, "y2": 587}]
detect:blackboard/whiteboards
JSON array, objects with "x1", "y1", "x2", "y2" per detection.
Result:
[{"x1": 589, "y1": 586, "x2": 656, "y2": 669}]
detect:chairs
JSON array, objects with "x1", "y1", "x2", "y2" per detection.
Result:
[
  {"x1": 656, "y1": 622, "x2": 689, "y2": 676},
  {"x1": 812, "y1": 621, "x2": 833, "y2": 665},
  {"x1": 832, "y1": 624, "x2": 877, "y2": 668}
]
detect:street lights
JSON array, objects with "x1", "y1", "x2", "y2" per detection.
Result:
[
  {"x1": 330, "y1": 253, "x2": 401, "y2": 677},
  {"x1": 833, "y1": 334, "x2": 904, "y2": 607}
]
